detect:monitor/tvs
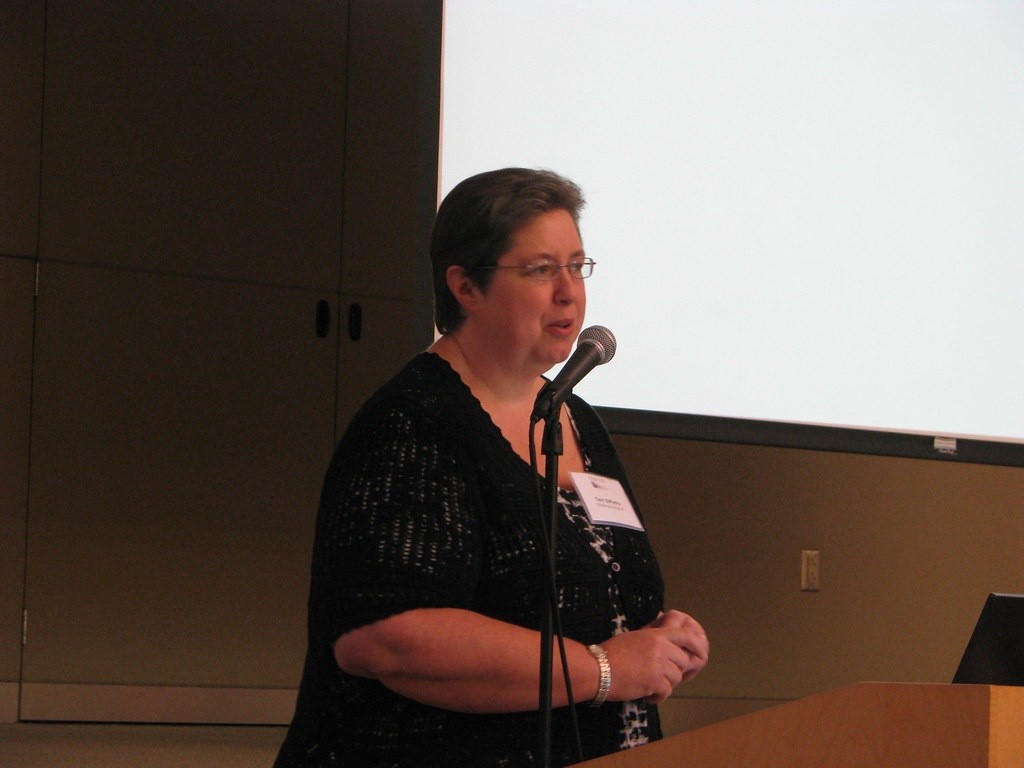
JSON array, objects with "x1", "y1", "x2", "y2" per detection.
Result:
[{"x1": 952, "y1": 592, "x2": 1024, "y2": 687}]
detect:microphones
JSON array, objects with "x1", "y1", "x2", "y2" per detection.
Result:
[{"x1": 530, "y1": 326, "x2": 617, "y2": 423}]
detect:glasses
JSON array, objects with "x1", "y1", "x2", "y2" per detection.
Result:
[{"x1": 464, "y1": 257, "x2": 597, "y2": 283}]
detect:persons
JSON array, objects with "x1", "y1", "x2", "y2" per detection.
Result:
[{"x1": 273, "y1": 166, "x2": 711, "y2": 768}]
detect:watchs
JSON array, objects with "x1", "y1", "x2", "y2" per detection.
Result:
[{"x1": 588, "y1": 644, "x2": 612, "y2": 704}]
